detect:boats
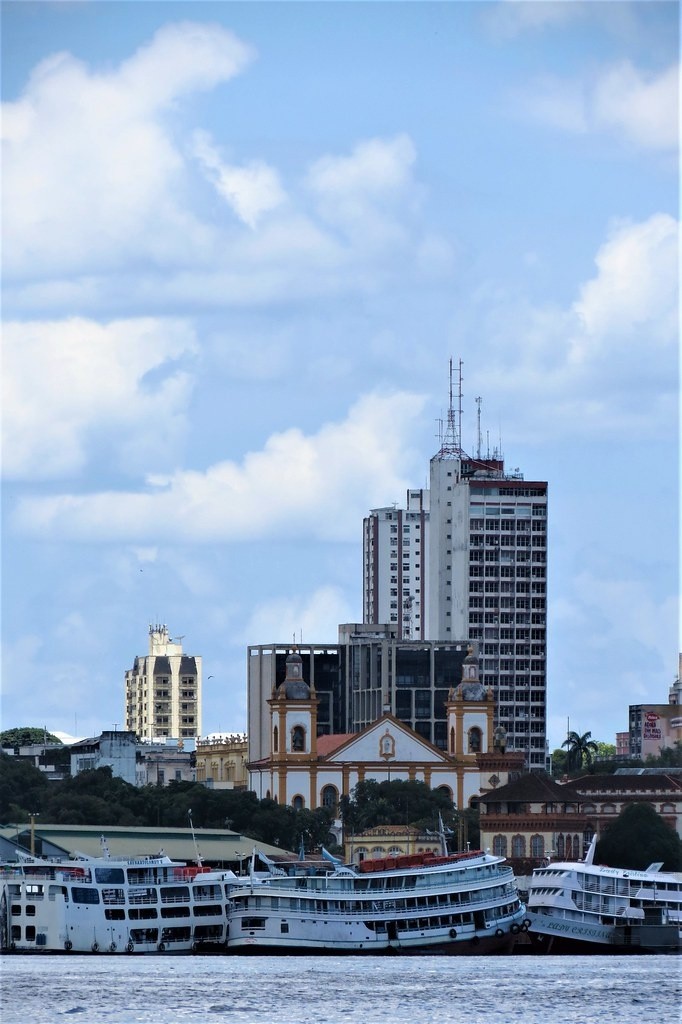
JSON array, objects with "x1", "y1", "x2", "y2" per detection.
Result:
[
  {"x1": 0, "y1": 809, "x2": 237, "y2": 956},
  {"x1": 227, "y1": 810, "x2": 526, "y2": 956},
  {"x1": 526, "y1": 832, "x2": 682, "y2": 954}
]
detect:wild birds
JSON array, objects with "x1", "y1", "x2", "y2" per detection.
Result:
[{"x1": 207, "y1": 675, "x2": 213, "y2": 679}]
